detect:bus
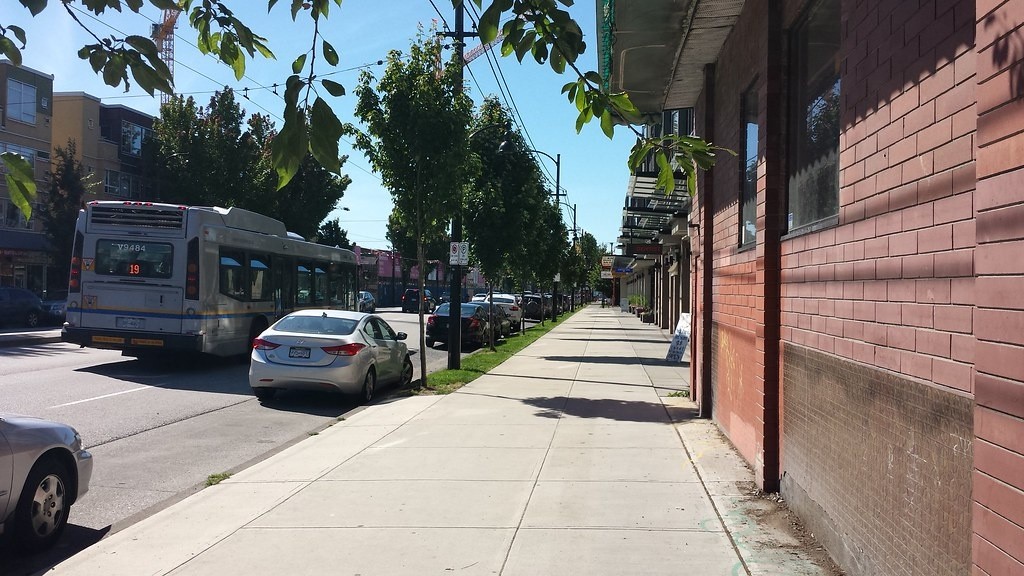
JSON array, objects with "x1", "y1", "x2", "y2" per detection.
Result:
[{"x1": 59, "y1": 199, "x2": 372, "y2": 359}]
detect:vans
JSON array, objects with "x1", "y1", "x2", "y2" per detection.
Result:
[{"x1": 402, "y1": 289, "x2": 436, "y2": 313}]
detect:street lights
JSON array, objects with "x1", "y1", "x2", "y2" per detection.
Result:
[
  {"x1": 517, "y1": 147, "x2": 562, "y2": 321},
  {"x1": 446, "y1": 120, "x2": 519, "y2": 372}
]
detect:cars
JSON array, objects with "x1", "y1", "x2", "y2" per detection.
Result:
[
  {"x1": 483, "y1": 294, "x2": 523, "y2": 331},
  {"x1": 248, "y1": 308, "x2": 414, "y2": 403},
  {"x1": 425, "y1": 302, "x2": 489, "y2": 348},
  {"x1": 515, "y1": 290, "x2": 585, "y2": 320},
  {"x1": 0, "y1": 286, "x2": 46, "y2": 329},
  {"x1": 485, "y1": 291, "x2": 500, "y2": 299},
  {"x1": 358, "y1": 291, "x2": 376, "y2": 314},
  {"x1": 44, "y1": 288, "x2": 69, "y2": 322},
  {"x1": 0, "y1": 416, "x2": 94, "y2": 551},
  {"x1": 466, "y1": 302, "x2": 512, "y2": 342},
  {"x1": 471, "y1": 293, "x2": 487, "y2": 303}
]
